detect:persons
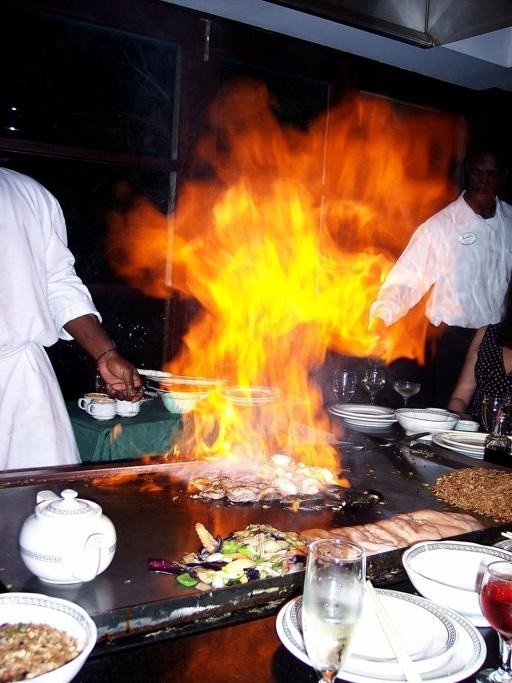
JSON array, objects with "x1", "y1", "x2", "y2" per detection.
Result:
[
  {"x1": 0, "y1": 167, "x2": 144, "y2": 470},
  {"x1": 448, "y1": 276, "x2": 512, "y2": 434},
  {"x1": 366, "y1": 147, "x2": 511, "y2": 376}
]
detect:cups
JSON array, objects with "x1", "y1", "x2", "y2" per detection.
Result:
[
  {"x1": 78, "y1": 392, "x2": 109, "y2": 415},
  {"x1": 454, "y1": 420, "x2": 480, "y2": 432},
  {"x1": 331, "y1": 368, "x2": 358, "y2": 405},
  {"x1": 114, "y1": 397, "x2": 141, "y2": 417},
  {"x1": 87, "y1": 399, "x2": 117, "y2": 420}
]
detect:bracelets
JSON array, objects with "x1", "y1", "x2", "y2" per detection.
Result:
[{"x1": 94, "y1": 342, "x2": 119, "y2": 364}]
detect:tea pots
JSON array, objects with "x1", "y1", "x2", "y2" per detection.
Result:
[{"x1": 19, "y1": 488, "x2": 117, "y2": 584}]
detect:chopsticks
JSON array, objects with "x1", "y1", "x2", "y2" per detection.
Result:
[{"x1": 363, "y1": 579, "x2": 423, "y2": 682}]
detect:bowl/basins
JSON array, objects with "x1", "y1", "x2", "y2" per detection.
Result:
[
  {"x1": 0, "y1": 591, "x2": 98, "y2": 683},
  {"x1": 161, "y1": 392, "x2": 200, "y2": 415},
  {"x1": 401, "y1": 540, "x2": 512, "y2": 627},
  {"x1": 394, "y1": 408, "x2": 460, "y2": 441},
  {"x1": 275, "y1": 586, "x2": 489, "y2": 683}
]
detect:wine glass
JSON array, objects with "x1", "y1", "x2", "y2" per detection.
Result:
[
  {"x1": 475, "y1": 560, "x2": 512, "y2": 683},
  {"x1": 393, "y1": 379, "x2": 421, "y2": 408},
  {"x1": 361, "y1": 368, "x2": 386, "y2": 406},
  {"x1": 302, "y1": 538, "x2": 366, "y2": 683}
]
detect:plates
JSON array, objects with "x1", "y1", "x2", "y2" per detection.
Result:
[
  {"x1": 327, "y1": 403, "x2": 398, "y2": 428},
  {"x1": 219, "y1": 384, "x2": 283, "y2": 408},
  {"x1": 432, "y1": 431, "x2": 512, "y2": 459}
]
